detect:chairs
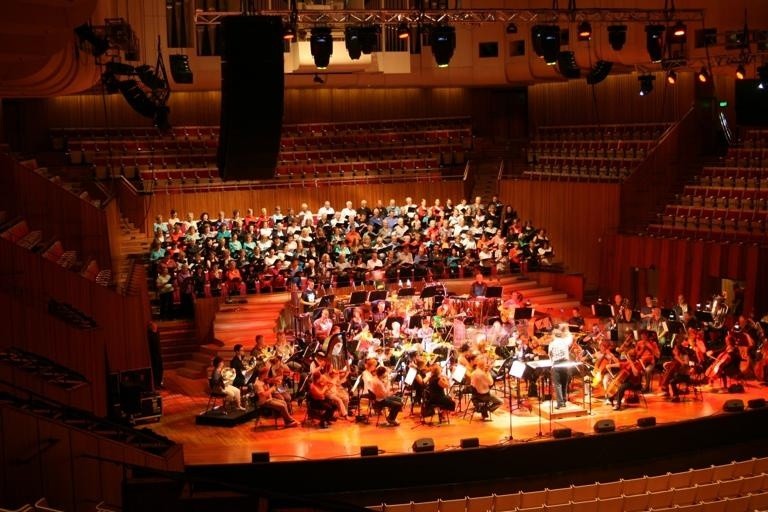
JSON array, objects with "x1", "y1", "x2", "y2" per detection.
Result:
[
  {"x1": 419, "y1": 388, "x2": 450, "y2": 425},
  {"x1": 623, "y1": 373, "x2": 648, "y2": 408},
  {"x1": 677, "y1": 364, "x2": 704, "y2": 402},
  {"x1": 249, "y1": 395, "x2": 281, "y2": 431},
  {"x1": 462, "y1": 384, "x2": 492, "y2": 423},
  {"x1": 366, "y1": 389, "x2": 390, "y2": 427},
  {"x1": 205, "y1": 377, "x2": 228, "y2": 415}
]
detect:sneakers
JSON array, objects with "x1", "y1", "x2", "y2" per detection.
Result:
[
  {"x1": 555, "y1": 404, "x2": 565, "y2": 409},
  {"x1": 388, "y1": 419, "x2": 399, "y2": 425},
  {"x1": 235, "y1": 406, "x2": 246, "y2": 411},
  {"x1": 612, "y1": 405, "x2": 619, "y2": 410},
  {"x1": 671, "y1": 396, "x2": 679, "y2": 401},
  {"x1": 286, "y1": 420, "x2": 301, "y2": 427}
]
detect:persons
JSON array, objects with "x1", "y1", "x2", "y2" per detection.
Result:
[{"x1": 147, "y1": 196, "x2": 768, "y2": 429}]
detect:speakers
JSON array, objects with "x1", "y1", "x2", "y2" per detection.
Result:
[
  {"x1": 217, "y1": 15, "x2": 285, "y2": 183},
  {"x1": 170, "y1": 54, "x2": 193, "y2": 84},
  {"x1": 734, "y1": 78, "x2": 768, "y2": 128}
]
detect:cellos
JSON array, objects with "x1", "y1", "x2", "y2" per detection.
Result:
[
  {"x1": 605, "y1": 360, "x2": 639, "y2": 399},
  {"x1": 591, "y1": 352, "x2": 610, "y2": 388},
  {"x1": 705, "y1": 348, "x2": 731, "y2": 379}
]
